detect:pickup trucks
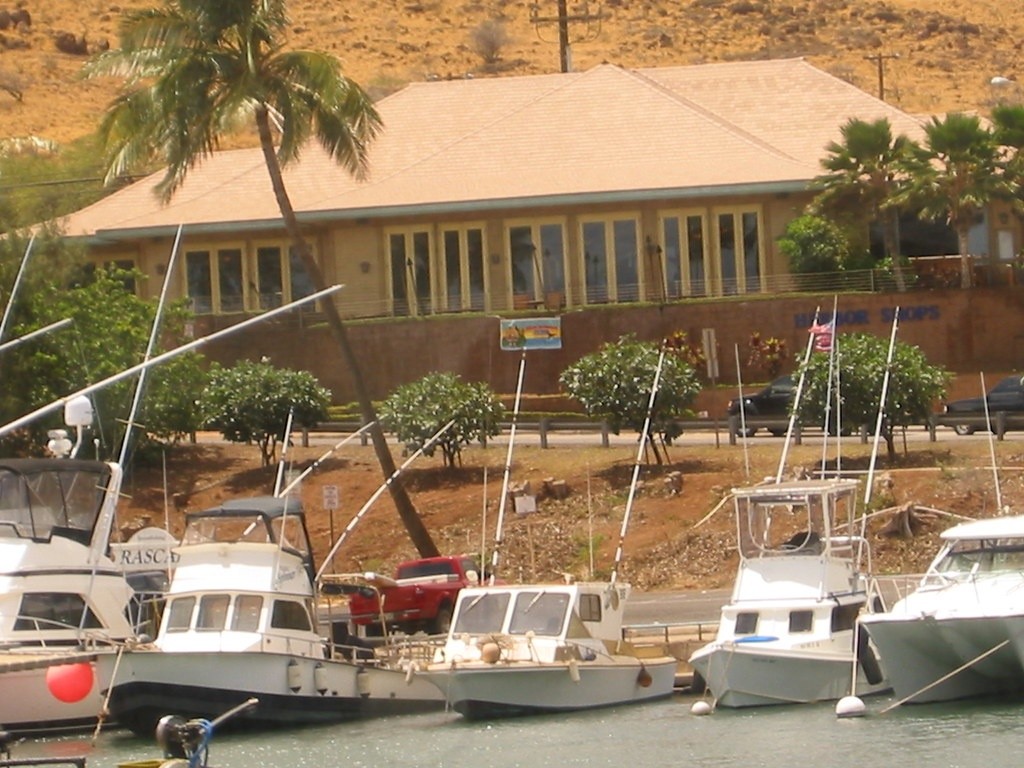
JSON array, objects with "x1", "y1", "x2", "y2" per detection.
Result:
[{"x1": 351, "y1": 553, "x2": 492, "y2": 637}]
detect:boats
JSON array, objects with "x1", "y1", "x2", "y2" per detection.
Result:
[
  {"x1": 410, "y1": 340, "x2": 678, "y2": 720},
  {"x1": 858, "y1": 373, "x2": 1024, "y2": 705},
  {"x1": 0, "y1": 219, "x2": 459, "y2": 742}
]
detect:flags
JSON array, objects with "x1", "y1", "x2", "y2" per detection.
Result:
[{"x1": 809, "y1": 321, "x2": 833, "y2": 351}]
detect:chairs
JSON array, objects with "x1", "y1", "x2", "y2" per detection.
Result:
[{"x1": 787, "y1": 531, "x2": 821, "y2": 556}]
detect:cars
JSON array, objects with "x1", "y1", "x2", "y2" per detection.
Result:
[
  {"x1": 942, "y1": 376, "x2": 1024, "y2": 436},
  {"x1": 726, "y1": 368, "x2": 875, "y2": 437}
]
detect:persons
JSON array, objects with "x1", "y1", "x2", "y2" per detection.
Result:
[{"x1": 27, "y1": 443, "x2": 94, "y2": 523}]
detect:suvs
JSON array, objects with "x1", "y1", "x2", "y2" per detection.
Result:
[{"x1": 687, "y1": 293, "x2": 902, "y2": 709}]
